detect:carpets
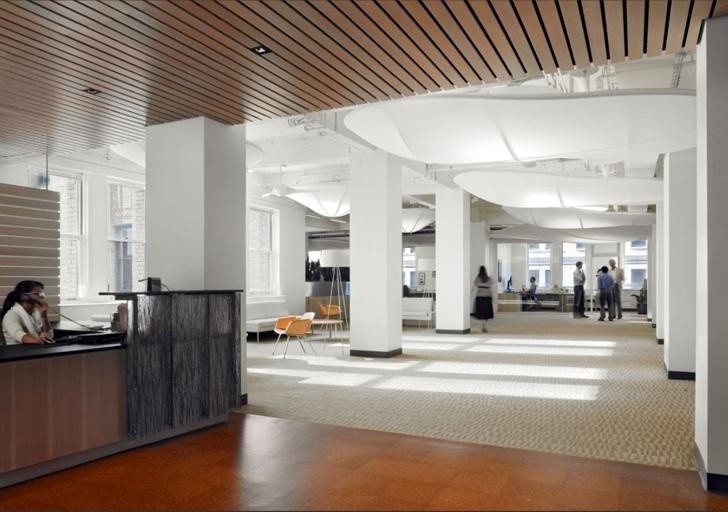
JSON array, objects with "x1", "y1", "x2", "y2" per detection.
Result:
[{"x1": 243, "y1": 307, "x2": 704, "y2": 471}]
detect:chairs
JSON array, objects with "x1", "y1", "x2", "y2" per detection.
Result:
[
  {"x1": 319, "y1": 302, "x2": 343, "y2": 318},
  {"x1": 272, "y1": 312, "x2": 316, "y2": 358}
]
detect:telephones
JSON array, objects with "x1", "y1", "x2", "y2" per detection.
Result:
[{"x1": 23, "y1": 294, "x2": 43, "y2": 307}]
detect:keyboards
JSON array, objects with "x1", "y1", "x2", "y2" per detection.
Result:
[{"x1": 55, "y1": 335, "x2": 79, "y2": 346}]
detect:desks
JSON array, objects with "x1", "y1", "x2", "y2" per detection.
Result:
[{"x1": 498, "y1": 292, "x2": 574, "y2": 312}]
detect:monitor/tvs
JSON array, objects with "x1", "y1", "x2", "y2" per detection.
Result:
[{"x1": 148, "y1": 277, "x2": 161, "y2": 292}]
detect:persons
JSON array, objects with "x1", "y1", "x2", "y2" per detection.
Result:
[
  {"x1": 522, "y1": 276, "x2": 537, "y2": 311},
  {"x1": 572, "y1": 261, "x2": 589, "y2": 319},
  {"x1": 608, "y1": 259, "x2": 625, "y2": 319},
  {"x1": 0, "y1": 280, "x2": 57, "y2": 346},
  {"x1": 597, "y1": 266, "x2": 614, "y2": 321},
  {"x1": 507, "y1": 275, "x2": 514, "y2": 291},
  {"x1": 470, "y1": 266, "x2": 497, "y2": 333}
]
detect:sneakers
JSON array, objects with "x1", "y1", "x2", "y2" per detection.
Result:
[{"x1": 572, "y1": 314, "x2": 622, "y2": 322}]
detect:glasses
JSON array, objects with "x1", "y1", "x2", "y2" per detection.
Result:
[{"x1": 23, "y1": 291, "x2": 45, "y2": 297}]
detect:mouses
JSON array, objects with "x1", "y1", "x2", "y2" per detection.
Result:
[{"x1": 44, "y1": 338, "x2": 56, "y2": 345}]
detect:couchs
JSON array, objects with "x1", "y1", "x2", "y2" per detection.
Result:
[
  {"x1": 246, "y1": 301, "x2": 293, "y2": 345},
  {"x1": 403, "y1": 296, "x2": 434, "y2": 328}
]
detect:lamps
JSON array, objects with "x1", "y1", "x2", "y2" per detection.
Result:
[
  {"x1": 417, "y1": 257, "x2": 437, "y2": 328},
  {"x1": 320, "y1": 248, "x2": 351, "y2": 331}
]
